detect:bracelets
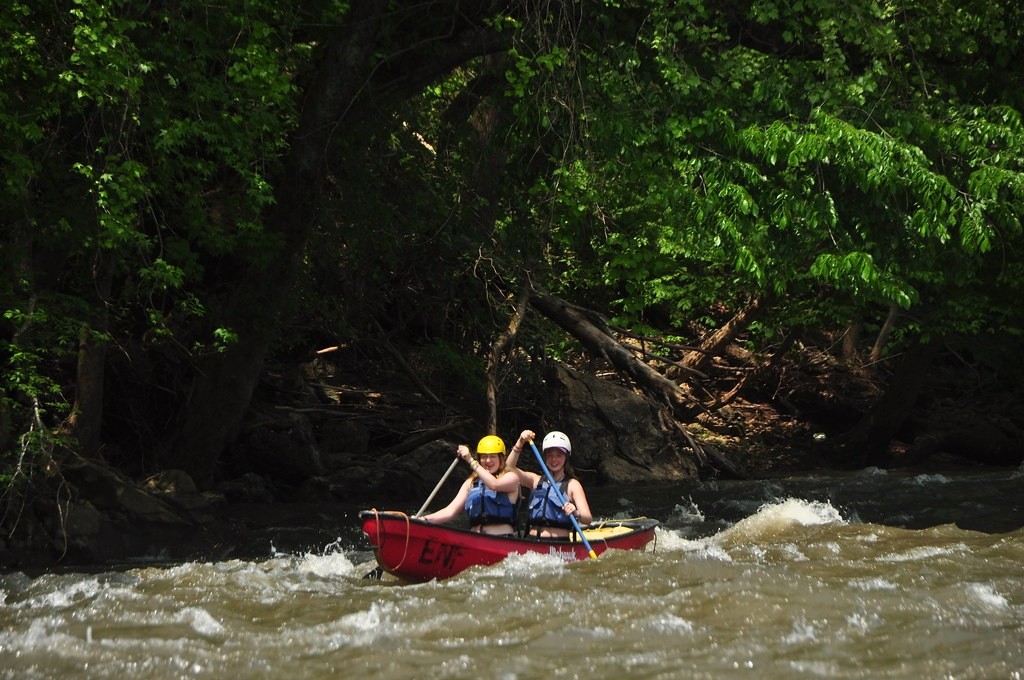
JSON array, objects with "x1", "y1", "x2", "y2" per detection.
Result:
[
  {"x1": 422, "y1": 515, "x2": 429, "y2": 523},
  {"x1": 512, "y1": 445, "x2": 522, "y2": 453},
  {"x1": 469, "y1": 459, "x2": 479, "y2": 471},
  {"x1": 576, "y1": 508, "x2": 582, "y2": 521}
]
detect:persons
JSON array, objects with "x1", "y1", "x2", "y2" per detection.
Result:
[
  {"x1": 506, "y1": 429, "x2": 592, "y2": 544},
  {"x1": 411, "y1": 434, "x2": 520, "y2": 540}
]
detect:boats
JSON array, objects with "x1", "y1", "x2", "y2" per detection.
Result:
[{"x1": 357, "y1": 509, "x2": 659, "y2": 582}]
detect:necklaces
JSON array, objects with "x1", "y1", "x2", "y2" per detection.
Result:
[{"x1": 555, "y1": 474, "x2": 565, "y2": 483}]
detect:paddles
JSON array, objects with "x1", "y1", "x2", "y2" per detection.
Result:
[
  {"x1": 412, "y1": 448, "x2": 466, "y2": 516},
  {"x1": 527, "y1": 439, "x2": 600, "y2": 560}
]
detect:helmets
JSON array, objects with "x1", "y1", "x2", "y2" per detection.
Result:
[
  {"x1": 477, "y1": 436, "x2": 506, "y2": 456},
  {"x1": 542, "y1": 431, "x2": 571, "y2": 456}
]
